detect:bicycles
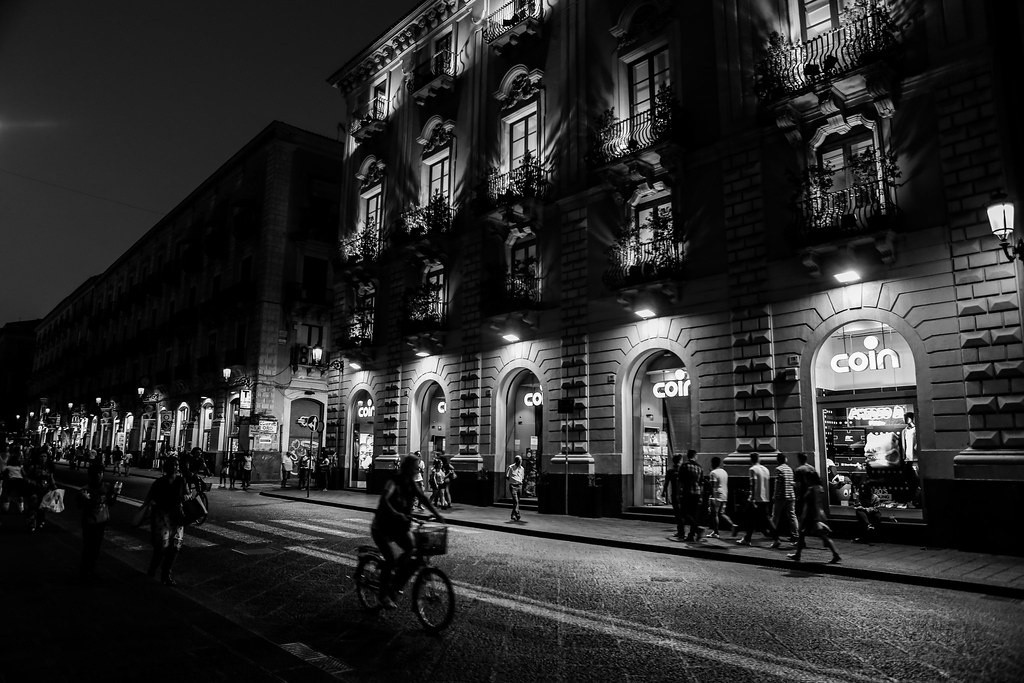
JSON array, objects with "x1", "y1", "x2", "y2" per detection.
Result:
[
  {"x1": 352, "y1": 514, "x2": 456, "y2": 634},
  {"x1": 180, "y1": 472, "x2": 212, "y2": 525}
]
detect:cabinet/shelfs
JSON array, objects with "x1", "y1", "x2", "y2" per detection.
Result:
[{"x1": 643, "y1": 426, "x2": 662, "y2": 474}]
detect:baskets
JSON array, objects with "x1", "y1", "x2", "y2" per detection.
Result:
[{"x1": 412, "y1": 526, "x2": 448, "y2": 556}]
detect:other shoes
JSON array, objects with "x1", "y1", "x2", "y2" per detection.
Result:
[
  {"x1": 792, "y1": 542, "x2": 806, "y2": 547},
  {"x1": 828, "y1": 554, "x2": 841, "y2": 563},
  {"x1": 732, "y1": 524, "x2": 739, "y2": 537},
  {"x1": 771, "y1": 540, "x2": 780, "y2": 548},
  {"x1": 696, "y1": 529, "x2": 705, "y2": 542},
  {"x1": 379, "y1": 596, "x2": 397, "y2": 609},
  {"x1": 416, "y1": 502, "x2": 452, "y2": 510},
  {"x1": 706, "y1": 531, "x2": 720, "y2": 538},
  {"x1": 787, "y1": 554, "x2": 800, "y2": 561},
  {"x1": 511, "y1": 517, "x2": 516, "y2": 521},
  {"x1": 672, "y1": 533, "x2": 684, "y2": 537},
  {"x1": 219, "y1": 484, "x2": 250, "y2": 490},
  {"x1": 735, "y1": 538, "x2": 751, "y2": 546},
  {"x1": 517, "y1": 515, "x2": 520, "y2": 521}
]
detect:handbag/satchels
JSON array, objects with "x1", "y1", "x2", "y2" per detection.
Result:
[
  {"x1": 96, "y1": 502, "x2": 110, "y2": 524},
  {"x1": 71, "y1": 490, "x2": 90, "y2": 520},
  {"x1": 133, "y1": 503, "x2": 152, "y2": 533},
  {"x1": 180, "y1": 494, "x2": 208, "y2": 524},
  {"x1": 39, "y1": 488, "x2": 64, "y2": 513}
]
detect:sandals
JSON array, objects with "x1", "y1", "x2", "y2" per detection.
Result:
[{"x1": 684, "y1": 537, "x2": 695, "y2": 542}]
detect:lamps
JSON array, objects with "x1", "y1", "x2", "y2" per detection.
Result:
[
  {"x1": 416, "y1": 347, "x2": 432, "y2": 358},
  {"x1": 348, "y1": 360, "x2": 363, "y2": 372},
  {"x1": 310, "y1": 342, "x2": 344, "y2": 372},
  {"x1": 501, "y1": 325, "x2": 523, "y2": 344},
  {"x1": 304, "y1": 391, "x2": 316, "y2": 395},
  {"x1": 137, "y1": 386, "x2": 159, "y2": 402},
  {"x1": 863, "y1": 335, "x2": 880, "y2": 350},
  {"x1": 628, "y1": 263, "x2": 652, "y2": 276},
  {"x1": 348, "y1": 253, "x2": 361, "y2": 263},
  {"x1": 222, "y1": 367, "x2": 250, "y2": 389},
  {"x1": 626, "y1": 138, "x2": 639, "y2": 152},
  {"x1": 632, "y1": 299, "x2": 658, "y2": 320},
  {"x1": 983, "y1": 191, "x2": 1024, "y2": 261},
  {"x1": 803, "y1": 64, "x2": 818, "y2": 80},
  {"x1": 832, "y1": 266, "x2": 864, "y2": 284}
]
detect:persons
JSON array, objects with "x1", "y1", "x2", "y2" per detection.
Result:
[
  {"x1": 705, "y1": 457, "x2": 739, "y2": 538},
  {"x1": 770, "y1": 454, "x2": 798, "y2": 531},
  {"x1": 0, "y1": 442, "x2": 258, "y2": 586},
  {"x1": 736, "y1": 453, "x2": 776, "y2": 546},
  {"x1": 787, "y1": 452, "x2": 842, "y2": 563},
  {"x1": 826, "y1": 459, "x2": 880, "y2": 542},
  {"x1": 280, "y1": 448, "x2": 338, "y2": 491},
  {"x1": 661, "y1": 455, "x2": 685, "y2": 541},
  {"x1": 372, "y1": 455, "x2": 445, "y2": 609},
  {"x1": 506, "y1": 455, "x2": 524, "y2": 521},
  {"x1": 412, "y1": 451, "x2": 454, "y2": 510},
  {"x1": 678, "y1": 450, "x2": 705, "y2": 541}
]
